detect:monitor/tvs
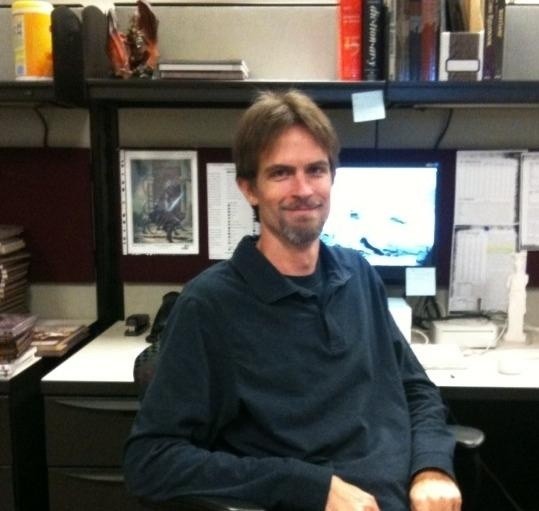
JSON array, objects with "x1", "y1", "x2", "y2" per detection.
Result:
[{"x1": 318, "y1": 161, "x2": 440, "y2": 283}]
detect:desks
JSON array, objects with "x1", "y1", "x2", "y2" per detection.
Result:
[{"x1": 38, "y1": 318, "x2": 538, "y2": 510}]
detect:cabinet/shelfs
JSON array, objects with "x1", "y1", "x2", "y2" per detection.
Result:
[
  {"x1": 0, "y1": 76, "x2": 86, "y2": 107},
  {"x1": 84, "y1": 69, "x2": 539, "y2": 327}
]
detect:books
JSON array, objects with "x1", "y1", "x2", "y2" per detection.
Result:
[
  {"x1": 0, "y1": 223, "x2": 88, "y2": 379},
  {"x1": 337, "y1": 0, "x2": 506, "y2": 81},
  {"x1": 158, "y1": 60, "x2": 248, "y2": 80}
]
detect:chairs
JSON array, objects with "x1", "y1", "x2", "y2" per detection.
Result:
[{"x1": 132, "y1": 340, "x2": 486, "y2": 511}]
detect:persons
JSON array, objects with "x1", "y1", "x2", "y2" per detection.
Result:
[{"x1": 122, "y1": 91, "x2": 462, "y2": 511}]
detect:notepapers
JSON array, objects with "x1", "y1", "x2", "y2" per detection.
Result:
[
  {"x1": 403, "y1": 266, "x2": 437, "y2": 297},
  {"x1": 346, "y1": 90, "x2": 390, "y2": 123}
]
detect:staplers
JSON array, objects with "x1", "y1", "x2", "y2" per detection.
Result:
[{"x1": 125, "y1": 314, "x2": 150, "y2": 337}]
